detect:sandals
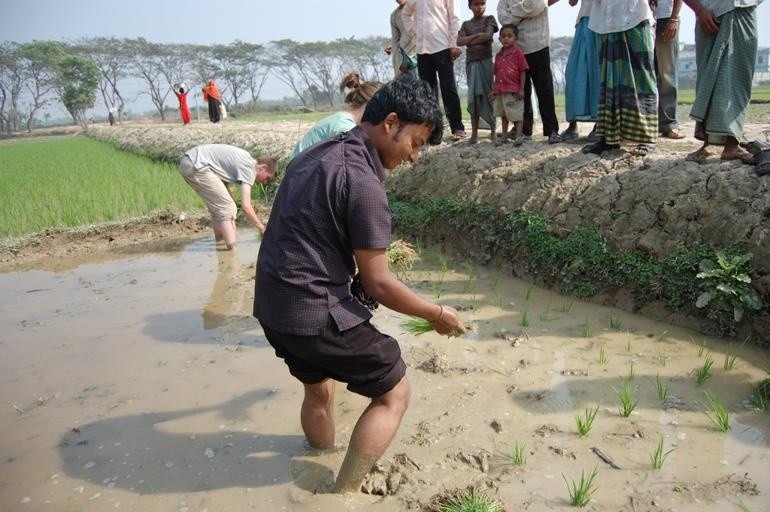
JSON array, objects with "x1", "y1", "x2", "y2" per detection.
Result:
[
  {"x1": 663, "y1": 129, "x2": 686, "y2": 138},
  {"x1": 514, "y1": 134, "x2": 523, "y2": 146},
  {"x1": 752, "y1": 149, "x2": 770, "y2": 175},
  {"x1": 684, "y1": 147, "x2": 721, "y2": 161},
  {"x1": 452, "y1": 129, "x2": 468, "y2": 141},
  {"x1": 721, "y1": 149, "x2": 759, "y2": 164},
  {"x1": 740, "y1": 139, "x2": 770, "y2": 155},
  {"x1": 494, "y1": 137, "x2": 507, "y2": 146}
]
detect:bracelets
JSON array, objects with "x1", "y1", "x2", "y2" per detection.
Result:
[{"x1": 427, "y1": 304, "x2": 445, "y2": 324}]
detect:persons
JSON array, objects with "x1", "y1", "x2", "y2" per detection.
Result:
[
  {"x1": 457, "y1": 1, "x2": 501, "y2": 146},
  {"x1": 384, "y1": 0, "x2": 466, "y2": 145},
  {"x1": 201, "y1": 79, "x2": 224, "y2": 124},
  {"x1": 170, "y1": 82, "x2": 197, "y2": 125},
  {"x1": 559, "y1": 1, "x2": 684, "y2": 156},
  {"x1": 108, "y1": 108, "x2": 115, "y2": 127},
  {"x1": 248, "y1": 72, "x2": 468, "y2": 498},
  {"x1": 287, "y1": 72, "x2": 387, "y2": 162},
  {"x1": 488, "y1": 24, "x2": 529, "y2": 146},
  {"x1": 179, "y1": 143, "x2": 281, "y2": 252},
  {"x1": 685, "y1": 1, "x2": 763, "y2": 163},
  {"x1": 496, "y1": 0, "x2": 561, "y2": 144}
]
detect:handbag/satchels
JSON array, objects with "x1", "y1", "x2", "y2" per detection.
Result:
[
  {"x1": 399, "y1": 50, "x2": 418, "y2": 74},
  {"x1": 218, "y1": 104, "x2": 227, "y2": 119}
]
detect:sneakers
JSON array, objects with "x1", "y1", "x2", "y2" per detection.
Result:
[
  {"x1": 548, "y1": 132, "x2": 562, "y2": 143},
  {"x1": 588, "y1": 127, "x2": 597, "y2": 141},
  {"x1": 561, "y1": 131, "x2": 578, "y2": 140},
  {"x1": 635, "y1": 143, "x2": 659, "y2": 155},
  {"x1": 508, "y1": 130, "x2": 532, "y2": 140},
  {"x1": 582, "y1": 140, "x2": 621, "y2": 154}
]
